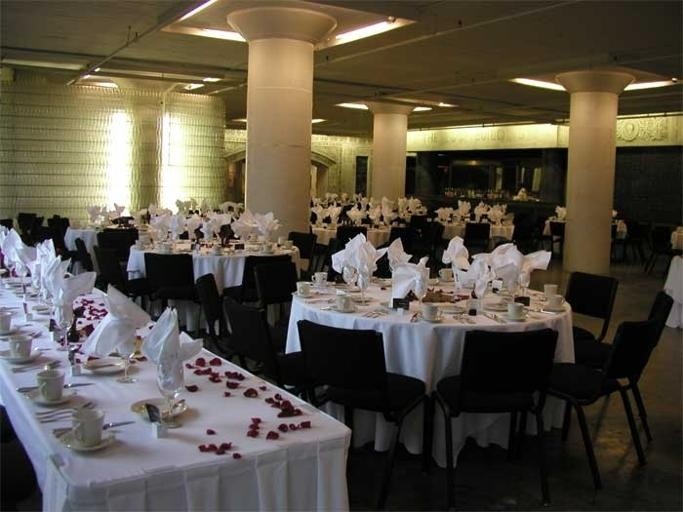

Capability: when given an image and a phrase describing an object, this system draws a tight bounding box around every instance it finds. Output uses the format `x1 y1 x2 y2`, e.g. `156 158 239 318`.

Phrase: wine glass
5 262 27 288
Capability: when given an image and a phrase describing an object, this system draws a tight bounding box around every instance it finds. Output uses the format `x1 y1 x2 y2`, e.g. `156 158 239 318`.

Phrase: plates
295 268 567 325
0 286 188 453
134 232 293 256
